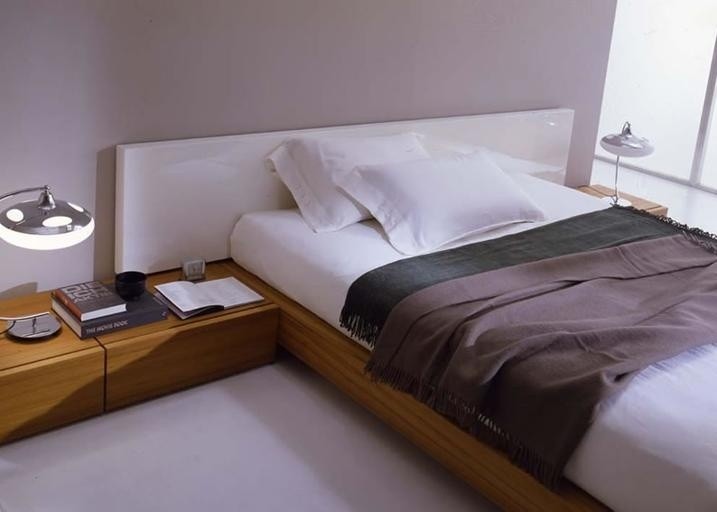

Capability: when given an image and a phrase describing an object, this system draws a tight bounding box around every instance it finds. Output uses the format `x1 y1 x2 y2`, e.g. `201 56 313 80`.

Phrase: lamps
0 185 96 339
598 121 654 207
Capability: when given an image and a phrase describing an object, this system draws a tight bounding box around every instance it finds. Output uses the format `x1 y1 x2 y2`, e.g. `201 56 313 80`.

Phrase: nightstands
571 184 668 221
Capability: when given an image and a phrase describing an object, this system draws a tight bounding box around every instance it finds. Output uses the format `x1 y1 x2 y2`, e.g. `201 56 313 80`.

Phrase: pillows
265 129 548 257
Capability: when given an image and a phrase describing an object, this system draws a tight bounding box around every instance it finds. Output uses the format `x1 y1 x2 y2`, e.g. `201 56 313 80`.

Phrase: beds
227 175 715 510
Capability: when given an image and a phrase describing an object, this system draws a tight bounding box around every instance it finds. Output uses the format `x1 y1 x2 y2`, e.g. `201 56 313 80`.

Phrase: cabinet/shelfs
0 307 279 446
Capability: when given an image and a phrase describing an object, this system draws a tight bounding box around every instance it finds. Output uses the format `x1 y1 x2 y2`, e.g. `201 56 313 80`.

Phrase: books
151 275 265 321
54 280 128 322
50 281 169 340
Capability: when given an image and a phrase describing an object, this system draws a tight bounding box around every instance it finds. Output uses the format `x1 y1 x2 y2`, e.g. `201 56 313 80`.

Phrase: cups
116 270 147 300
